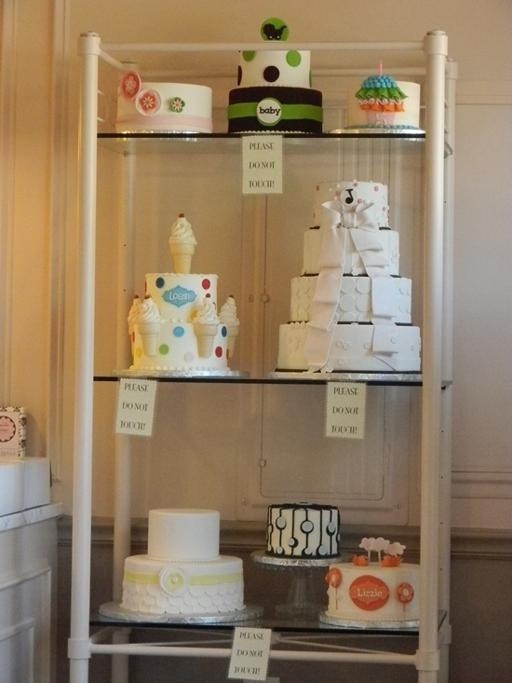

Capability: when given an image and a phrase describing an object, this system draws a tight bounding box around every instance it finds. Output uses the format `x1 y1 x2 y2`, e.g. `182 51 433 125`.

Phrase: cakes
228 17 323 133
120 508 246 614
275 181 421 374
115 70 213 133
344 63 420 129
127 214 240 370
325 537 420 621
264 502 341 559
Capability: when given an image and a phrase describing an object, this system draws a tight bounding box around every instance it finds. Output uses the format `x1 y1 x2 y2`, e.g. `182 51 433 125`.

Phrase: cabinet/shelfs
67 29 457 682
0 504 62 683
